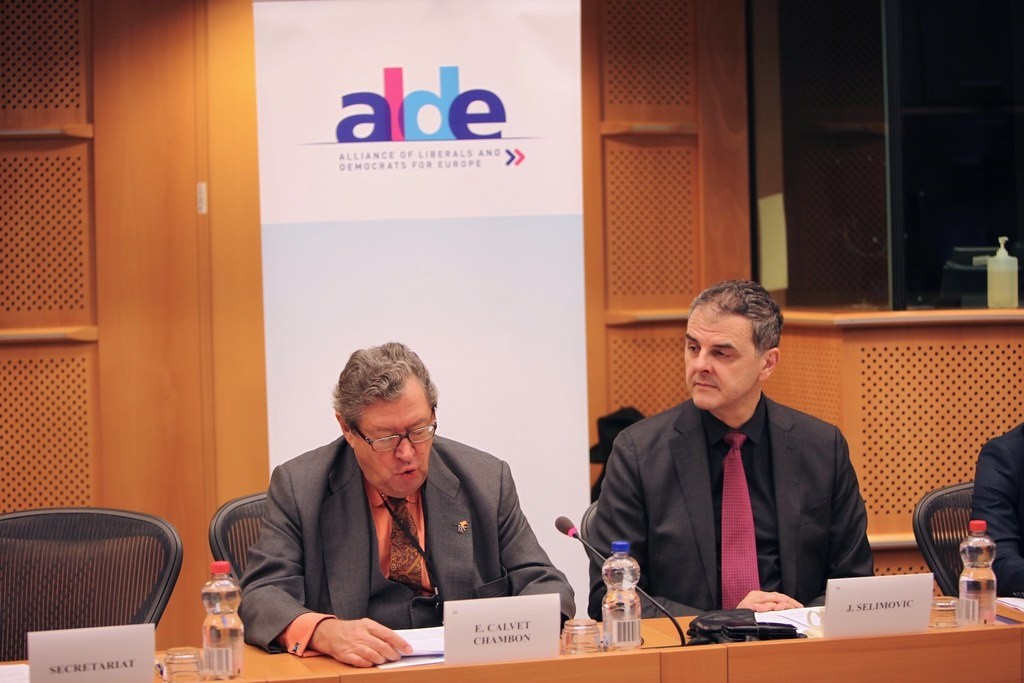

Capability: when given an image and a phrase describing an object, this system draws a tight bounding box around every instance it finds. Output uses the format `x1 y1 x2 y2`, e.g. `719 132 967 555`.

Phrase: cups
165 647 203 683
930 596 959 629
559 618 601 653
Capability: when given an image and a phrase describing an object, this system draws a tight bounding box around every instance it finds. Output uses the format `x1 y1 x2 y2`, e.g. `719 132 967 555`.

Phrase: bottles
957 521 997 628
601 540 642 651
201 561 244 679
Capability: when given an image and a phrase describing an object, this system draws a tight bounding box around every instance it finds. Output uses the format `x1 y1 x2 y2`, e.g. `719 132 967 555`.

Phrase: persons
238 342 576 668
971 422 1024 598
587 281 874 622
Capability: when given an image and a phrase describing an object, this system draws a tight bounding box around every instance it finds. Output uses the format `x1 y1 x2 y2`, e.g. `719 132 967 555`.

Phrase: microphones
555 516 686 647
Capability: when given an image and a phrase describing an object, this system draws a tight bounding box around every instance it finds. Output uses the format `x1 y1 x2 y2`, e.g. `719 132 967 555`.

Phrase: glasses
347 407 442 453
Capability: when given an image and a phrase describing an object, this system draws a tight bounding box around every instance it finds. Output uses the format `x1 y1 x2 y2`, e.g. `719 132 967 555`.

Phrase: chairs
912 480 974 599
0 507 184 662
208 491 268 583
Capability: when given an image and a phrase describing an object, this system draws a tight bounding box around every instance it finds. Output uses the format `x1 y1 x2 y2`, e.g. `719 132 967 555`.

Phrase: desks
641 600 1024 683
0 600 728 683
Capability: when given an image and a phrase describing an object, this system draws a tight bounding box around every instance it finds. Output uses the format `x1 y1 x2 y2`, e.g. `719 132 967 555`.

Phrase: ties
387 501 421 598
720 431 762 610
